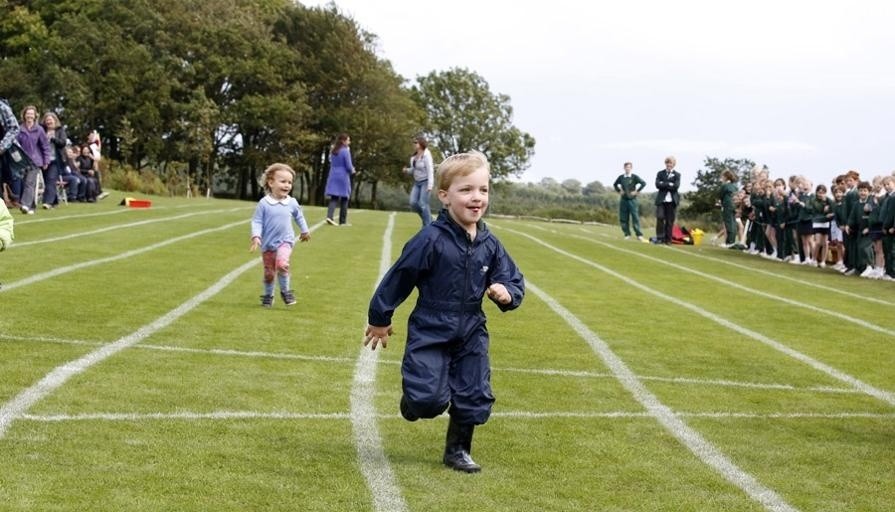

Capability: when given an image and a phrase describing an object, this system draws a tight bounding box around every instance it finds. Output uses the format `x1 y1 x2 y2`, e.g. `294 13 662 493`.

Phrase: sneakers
807 260 817 267
820 262 827 267
860 265 895 281
258 293 274 309
831 260 854 276
801 257 810 264
281 290 296 306
21 205 30 212
43 203 52 209
624 235 629 240
789 255 800 264
718 242 789 260
97 192 110 200
637 235 649 243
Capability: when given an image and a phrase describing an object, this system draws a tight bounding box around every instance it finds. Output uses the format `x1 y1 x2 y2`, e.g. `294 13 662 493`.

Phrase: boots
396 395 416 419
442 419 480 471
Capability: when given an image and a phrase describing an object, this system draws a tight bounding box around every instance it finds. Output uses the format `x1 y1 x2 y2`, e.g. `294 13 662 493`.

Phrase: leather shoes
666 240 672 247
68 196 77 202
341 223 351 227
326 218 338 227
79 196 87 202
654 239 664 244
87 196 96 202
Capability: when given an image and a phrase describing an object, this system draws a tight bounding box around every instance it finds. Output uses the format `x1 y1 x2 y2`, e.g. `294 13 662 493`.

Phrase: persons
365 150 527 476
0 97 20 208
764 179 786 258
60 131 110 201
812 183 834 268
612 162 647 242
0 196 18 253
16 104 51 215
829 170 873 276
321 133 357 227
871 176 884 279
790 172 808 264
402 138 435 232
794 176 816 266
882 176 895 281
654 156 678 245
775 187 791 260
715 169 737 249
733 168 774 255
41 110 67 210
785 176 799 264
249 163 311 309
